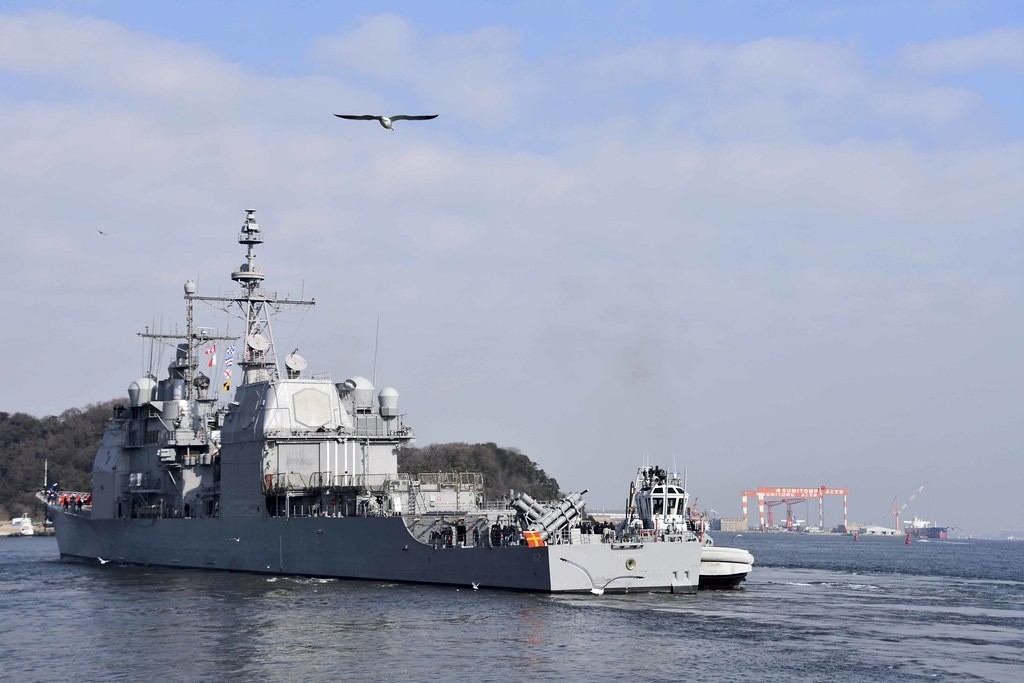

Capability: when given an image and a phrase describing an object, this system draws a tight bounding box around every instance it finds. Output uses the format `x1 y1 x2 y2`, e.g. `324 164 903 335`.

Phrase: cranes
890 477 928 531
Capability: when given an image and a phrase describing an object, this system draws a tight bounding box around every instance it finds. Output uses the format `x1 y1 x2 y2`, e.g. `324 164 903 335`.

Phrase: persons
503 524 516 543
63 495 83 511
473 526 481 546
578 521 614 534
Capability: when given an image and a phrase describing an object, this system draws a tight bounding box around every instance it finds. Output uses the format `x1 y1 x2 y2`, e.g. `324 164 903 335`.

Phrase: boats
36 206 755 595
610 450 713 545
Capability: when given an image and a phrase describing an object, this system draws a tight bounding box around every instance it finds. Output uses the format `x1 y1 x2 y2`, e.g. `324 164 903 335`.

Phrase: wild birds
401 544 409 553
97 557 111 566
472 581 481 590
327 109 446 135
727 533 745 544
228 535 242 544
95 227 110 238
559 557 644 598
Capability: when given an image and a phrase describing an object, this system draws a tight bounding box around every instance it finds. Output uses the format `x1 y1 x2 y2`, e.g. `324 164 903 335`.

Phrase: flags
525 530 544 548
205 343 216 367
222 345 235 392
